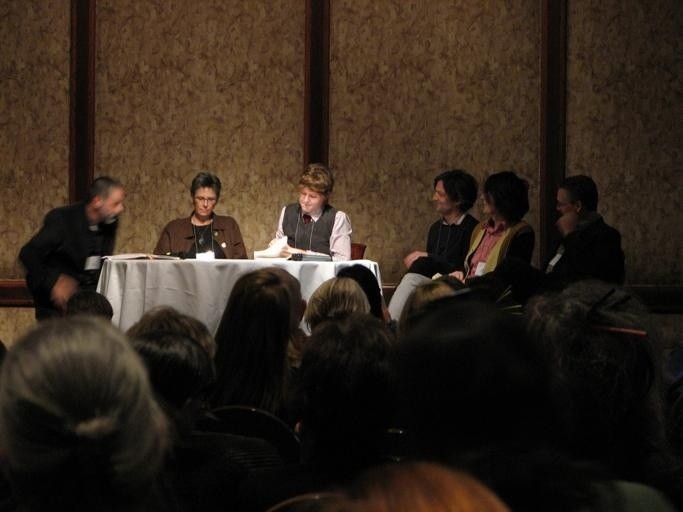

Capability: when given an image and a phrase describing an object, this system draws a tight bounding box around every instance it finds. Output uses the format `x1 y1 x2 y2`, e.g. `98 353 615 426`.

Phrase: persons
19 176 126 321
449 171 536 287
386 168 481 322
1 263 682 511
151 171 250 258
267 163 354 264
512 174 625 305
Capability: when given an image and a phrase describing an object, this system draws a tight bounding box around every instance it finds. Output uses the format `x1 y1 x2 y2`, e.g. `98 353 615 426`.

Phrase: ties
303 215 311 224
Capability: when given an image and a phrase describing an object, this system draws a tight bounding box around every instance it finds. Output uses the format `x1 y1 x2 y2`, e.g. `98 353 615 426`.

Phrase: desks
96 252 382 340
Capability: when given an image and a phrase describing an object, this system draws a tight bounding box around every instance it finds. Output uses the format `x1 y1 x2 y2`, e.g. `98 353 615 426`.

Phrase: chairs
351 243 367 259
495 245 567 316
200 404 304 470
265 491 347 511
